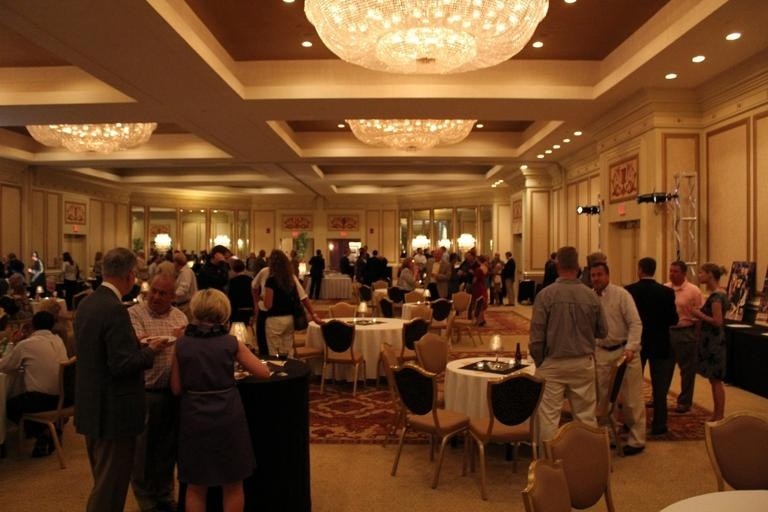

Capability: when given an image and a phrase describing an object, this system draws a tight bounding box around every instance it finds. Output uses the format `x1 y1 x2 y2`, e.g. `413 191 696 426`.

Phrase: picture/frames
281 214 314 231
512 198 522 221
608 153 639 205
65 200 87 226
327 214 360 231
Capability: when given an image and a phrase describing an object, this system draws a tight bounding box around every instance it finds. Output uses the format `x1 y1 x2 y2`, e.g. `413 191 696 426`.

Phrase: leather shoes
675 404 689 412
647 426 666 436
646 396 654 407
623 445 642 456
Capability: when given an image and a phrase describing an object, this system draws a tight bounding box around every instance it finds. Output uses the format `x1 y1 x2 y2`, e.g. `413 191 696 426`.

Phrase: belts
596 340 628 351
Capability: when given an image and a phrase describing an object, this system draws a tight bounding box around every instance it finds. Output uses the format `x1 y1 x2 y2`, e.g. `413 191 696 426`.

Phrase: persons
1 244 104 462
528 244 647 463
610 257 728 433
729 264 751 317
339 245 516 327
72 246 325 511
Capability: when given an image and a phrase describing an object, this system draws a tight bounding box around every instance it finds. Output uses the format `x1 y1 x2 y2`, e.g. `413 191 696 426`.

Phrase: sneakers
32 440 55 456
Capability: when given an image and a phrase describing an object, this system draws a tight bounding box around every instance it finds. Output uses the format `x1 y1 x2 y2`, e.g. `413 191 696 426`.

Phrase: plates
140 335 177 345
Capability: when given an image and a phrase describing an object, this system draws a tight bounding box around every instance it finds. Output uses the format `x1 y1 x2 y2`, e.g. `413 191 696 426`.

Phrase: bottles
514 342 521 369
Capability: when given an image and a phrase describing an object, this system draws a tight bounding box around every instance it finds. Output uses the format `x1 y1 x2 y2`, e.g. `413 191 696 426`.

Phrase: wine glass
276 347 289 376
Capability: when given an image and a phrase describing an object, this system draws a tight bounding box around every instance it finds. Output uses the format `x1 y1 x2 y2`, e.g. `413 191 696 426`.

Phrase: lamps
303 0 549 76
577 205 599 215
343 119 478 150
636 191 680 206
25 121 159 153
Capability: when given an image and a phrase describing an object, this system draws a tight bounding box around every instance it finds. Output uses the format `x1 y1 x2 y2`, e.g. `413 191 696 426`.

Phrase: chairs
292 279 491 404
522 452 572 511
470 371 549 501
540 419 617 511
703 408 768 492
0 277 144 470
386 361 471 490
563 352 631 470
